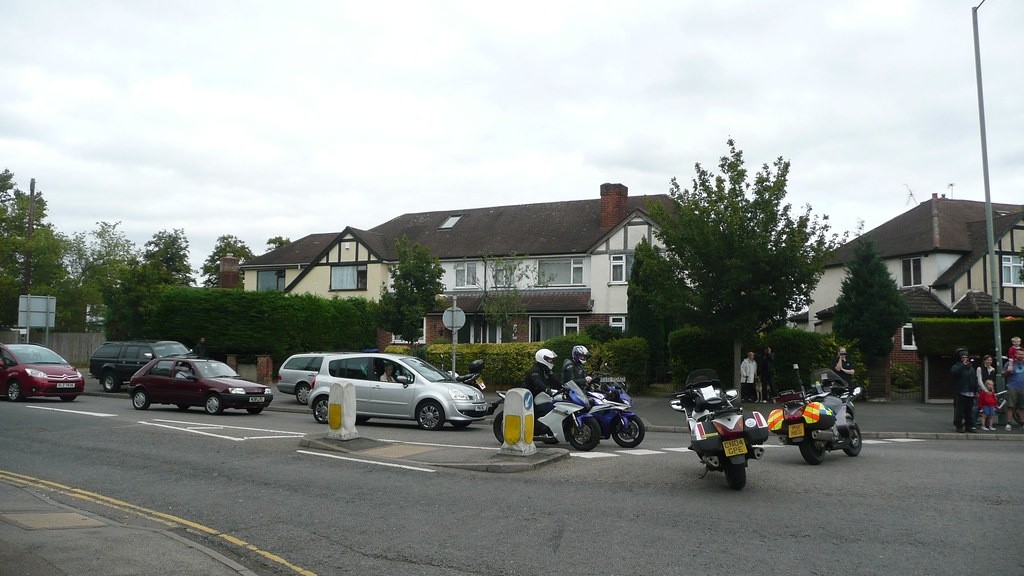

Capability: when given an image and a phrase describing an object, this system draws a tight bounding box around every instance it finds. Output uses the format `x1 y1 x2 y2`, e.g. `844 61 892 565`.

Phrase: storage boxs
468 359 484 373
802 402 837 431
767 408 788 435
742 410 769 444
691 421 723 452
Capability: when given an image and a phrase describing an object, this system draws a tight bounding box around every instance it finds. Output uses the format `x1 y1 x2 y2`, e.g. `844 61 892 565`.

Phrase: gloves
586 376 592 383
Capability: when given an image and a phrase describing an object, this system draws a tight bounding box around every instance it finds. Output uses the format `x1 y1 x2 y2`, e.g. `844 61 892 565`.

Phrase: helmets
572 345 590 367
534 348 558 371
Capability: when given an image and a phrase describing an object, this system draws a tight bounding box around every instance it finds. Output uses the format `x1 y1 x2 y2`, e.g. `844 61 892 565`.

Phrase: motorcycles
668 367 769 489
550 371 646 449
493 379 601 451
440 353 486 390
768 363 863 464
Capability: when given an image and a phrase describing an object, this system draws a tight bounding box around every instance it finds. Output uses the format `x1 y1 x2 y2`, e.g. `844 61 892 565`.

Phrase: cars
308 353 487 431
129 357 273 415
0 343 86 401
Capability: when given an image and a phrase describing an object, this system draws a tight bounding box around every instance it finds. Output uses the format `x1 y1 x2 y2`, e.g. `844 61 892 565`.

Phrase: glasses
839 353 847 355
961 357 968 358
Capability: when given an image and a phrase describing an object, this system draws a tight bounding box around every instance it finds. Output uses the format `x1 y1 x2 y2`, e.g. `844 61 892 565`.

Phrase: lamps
345 243 350 249
513 323 518 333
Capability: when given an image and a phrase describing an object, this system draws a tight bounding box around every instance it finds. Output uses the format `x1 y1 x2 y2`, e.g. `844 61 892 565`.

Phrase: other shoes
980 426 989 431
956 428 964 433
1005 423 1012 431
965 425 978 433
988 426 996 430
971 426 977 429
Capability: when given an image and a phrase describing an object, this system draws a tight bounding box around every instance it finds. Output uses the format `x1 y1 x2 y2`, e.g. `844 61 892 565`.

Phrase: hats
961 351 968 356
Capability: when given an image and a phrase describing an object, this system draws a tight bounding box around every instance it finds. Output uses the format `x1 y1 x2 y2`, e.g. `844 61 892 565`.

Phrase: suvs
277 353 326 405
90 339 199 393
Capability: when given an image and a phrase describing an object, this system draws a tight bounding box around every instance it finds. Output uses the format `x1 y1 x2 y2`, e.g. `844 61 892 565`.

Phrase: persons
520 348 563 406
759 347 777 404
175 362 194 379
380 364 396 382
1008 336 1024 374
561 345 592 400
978 380 997 431
976 355 995 392
832 348 855 388
951 351 977 433
1001 349 1024 431
741 352 758 403
